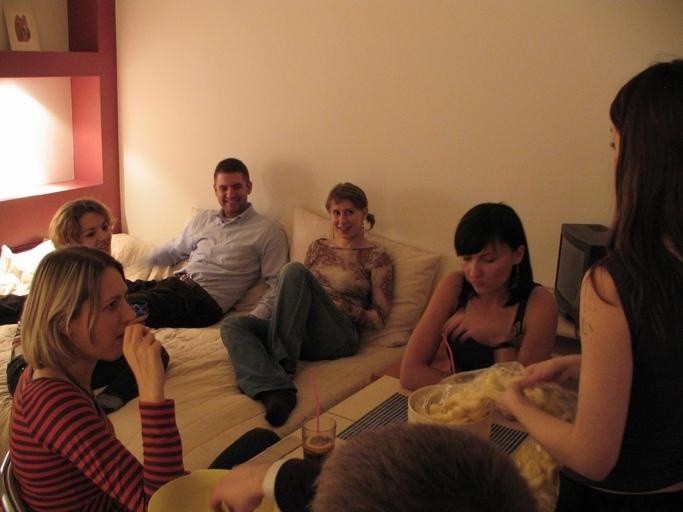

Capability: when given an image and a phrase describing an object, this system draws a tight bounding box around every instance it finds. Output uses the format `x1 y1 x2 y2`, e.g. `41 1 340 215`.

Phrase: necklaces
64 369 103 417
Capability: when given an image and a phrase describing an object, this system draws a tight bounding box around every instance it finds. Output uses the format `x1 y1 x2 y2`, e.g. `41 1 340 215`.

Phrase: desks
232 371 530 511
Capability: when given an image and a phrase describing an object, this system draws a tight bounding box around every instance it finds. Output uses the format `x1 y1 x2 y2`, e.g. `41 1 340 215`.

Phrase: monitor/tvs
553 224 611 330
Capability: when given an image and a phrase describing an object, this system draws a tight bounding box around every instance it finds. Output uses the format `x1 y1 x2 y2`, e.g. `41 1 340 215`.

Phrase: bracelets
493 341 514 351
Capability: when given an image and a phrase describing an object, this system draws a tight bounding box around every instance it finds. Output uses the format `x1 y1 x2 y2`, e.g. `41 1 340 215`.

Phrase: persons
8 246 280 512
0 159 288 329
6 198 169 415
495 59 683 512
220 182 395 427
399 202 559 391
209 422 538 512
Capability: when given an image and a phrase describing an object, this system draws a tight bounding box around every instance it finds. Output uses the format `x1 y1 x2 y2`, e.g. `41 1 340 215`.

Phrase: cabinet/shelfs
1 2 121 253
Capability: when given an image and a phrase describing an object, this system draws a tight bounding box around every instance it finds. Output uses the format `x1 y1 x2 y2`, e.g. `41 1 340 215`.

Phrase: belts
179 273 222 317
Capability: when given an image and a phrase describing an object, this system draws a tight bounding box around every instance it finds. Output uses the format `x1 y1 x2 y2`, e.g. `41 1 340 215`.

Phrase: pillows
290 204 441 349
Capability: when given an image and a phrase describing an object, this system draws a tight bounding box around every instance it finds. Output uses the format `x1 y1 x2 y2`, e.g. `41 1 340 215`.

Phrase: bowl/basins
407 384 492 441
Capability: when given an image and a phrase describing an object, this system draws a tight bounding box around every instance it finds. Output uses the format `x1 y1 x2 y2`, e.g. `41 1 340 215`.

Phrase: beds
1 323 405 476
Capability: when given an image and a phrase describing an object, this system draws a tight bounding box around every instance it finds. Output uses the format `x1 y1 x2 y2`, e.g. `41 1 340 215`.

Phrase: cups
301 415 336 462
